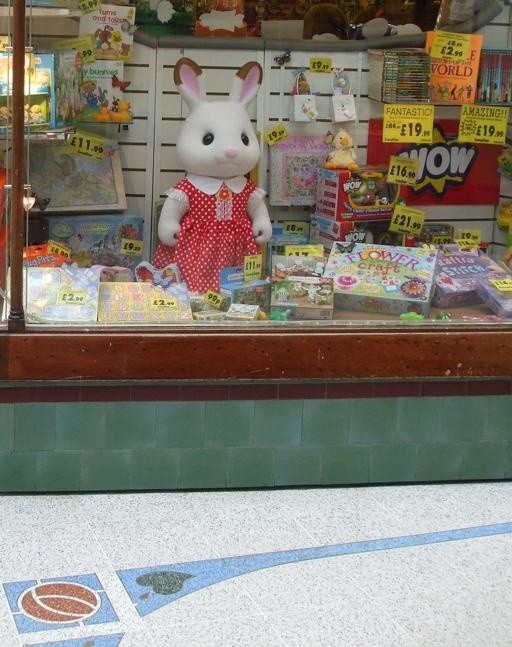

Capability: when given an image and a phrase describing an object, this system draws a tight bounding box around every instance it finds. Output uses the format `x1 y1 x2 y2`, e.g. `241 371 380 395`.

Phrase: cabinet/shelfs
0 303 512 494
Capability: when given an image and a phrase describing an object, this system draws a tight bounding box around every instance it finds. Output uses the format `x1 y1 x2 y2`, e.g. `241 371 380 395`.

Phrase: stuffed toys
326 129 359 171
295 75 310 96
332 72 350 96
0 103 44 127
134 58 274 294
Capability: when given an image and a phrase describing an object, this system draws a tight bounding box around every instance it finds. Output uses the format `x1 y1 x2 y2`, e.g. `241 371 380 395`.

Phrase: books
368 29 511 107
